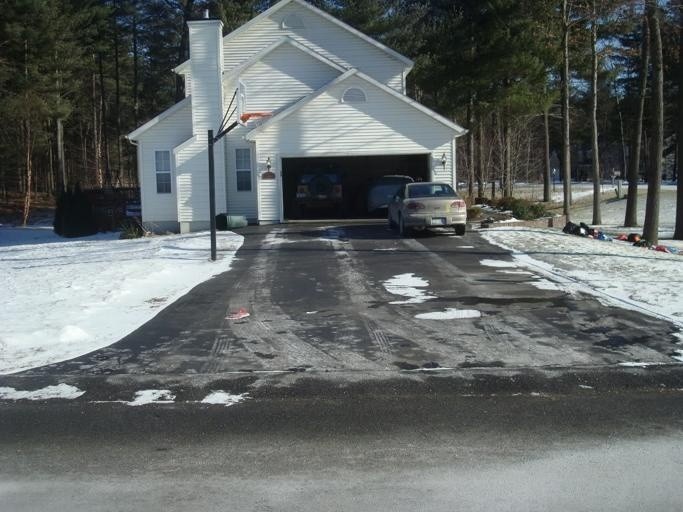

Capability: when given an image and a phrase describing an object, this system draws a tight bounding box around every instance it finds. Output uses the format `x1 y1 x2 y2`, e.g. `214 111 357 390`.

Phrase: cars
366 173 414 216
386 179 467 236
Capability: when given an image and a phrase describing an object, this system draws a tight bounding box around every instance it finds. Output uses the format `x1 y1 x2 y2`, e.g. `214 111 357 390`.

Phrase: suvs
296 161 344 216
